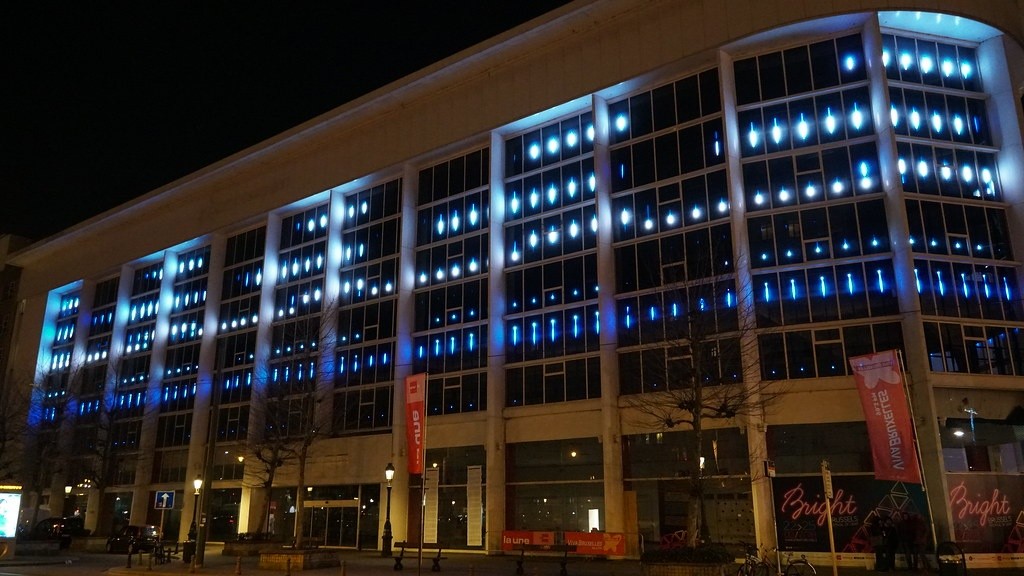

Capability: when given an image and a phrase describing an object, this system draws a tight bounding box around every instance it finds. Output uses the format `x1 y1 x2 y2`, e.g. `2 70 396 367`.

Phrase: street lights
52 485 73 541
700 456 713 547
381 462 396 555
186 475 203 541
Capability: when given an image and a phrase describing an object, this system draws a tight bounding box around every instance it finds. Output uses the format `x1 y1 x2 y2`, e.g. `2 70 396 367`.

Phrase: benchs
510 544 578 574
391 540 449 571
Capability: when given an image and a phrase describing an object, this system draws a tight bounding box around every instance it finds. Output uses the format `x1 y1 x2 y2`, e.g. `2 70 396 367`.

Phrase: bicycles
750 543 817 576
736 539 764 576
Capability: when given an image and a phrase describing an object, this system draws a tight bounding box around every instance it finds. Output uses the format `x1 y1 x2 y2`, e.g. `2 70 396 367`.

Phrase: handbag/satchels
871 535 888 547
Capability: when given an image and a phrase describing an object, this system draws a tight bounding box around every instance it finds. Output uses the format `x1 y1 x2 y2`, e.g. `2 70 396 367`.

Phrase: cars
105 524 164 554
28 516 84 539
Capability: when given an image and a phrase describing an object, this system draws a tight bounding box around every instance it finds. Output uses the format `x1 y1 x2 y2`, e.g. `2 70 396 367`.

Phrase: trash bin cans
874 544 896 572
182 540 196 563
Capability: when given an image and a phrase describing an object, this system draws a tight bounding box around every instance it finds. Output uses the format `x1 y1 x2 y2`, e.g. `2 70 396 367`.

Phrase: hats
871 516 881 522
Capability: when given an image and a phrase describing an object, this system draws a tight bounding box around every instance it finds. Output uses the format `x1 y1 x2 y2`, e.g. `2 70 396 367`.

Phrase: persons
189 524 198 557
900 512 919 572
870 516 887 573
882 517 898 571
916 513 931 576
